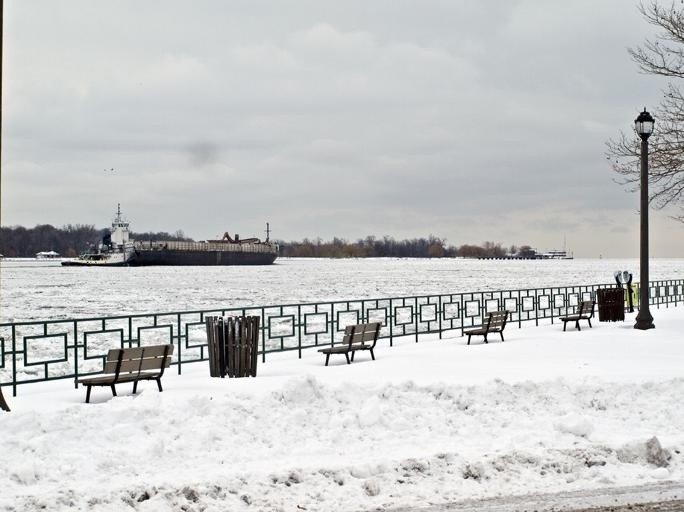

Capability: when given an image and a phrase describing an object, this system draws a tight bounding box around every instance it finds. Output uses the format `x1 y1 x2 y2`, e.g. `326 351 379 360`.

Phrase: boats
36 251 59 261
61 203 279 265
0 254 3 260
505 246 573 259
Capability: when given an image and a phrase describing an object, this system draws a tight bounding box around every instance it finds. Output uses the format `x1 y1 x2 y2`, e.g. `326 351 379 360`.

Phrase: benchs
462 310 509 345
317 322 382 366
75 344 175 403
559 301 596 331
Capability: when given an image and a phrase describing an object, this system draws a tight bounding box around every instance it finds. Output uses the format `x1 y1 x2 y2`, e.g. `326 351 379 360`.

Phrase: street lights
634 113 655 330
